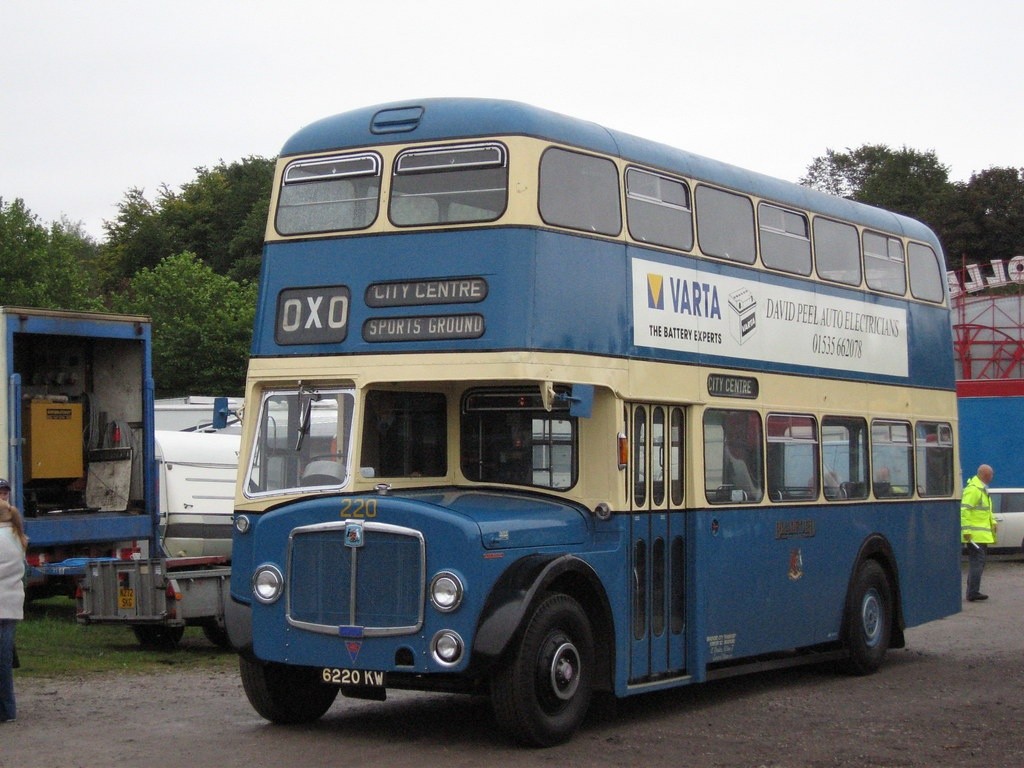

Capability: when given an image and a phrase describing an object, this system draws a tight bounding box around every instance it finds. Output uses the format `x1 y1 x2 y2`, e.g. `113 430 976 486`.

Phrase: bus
207 97 968 748
74 385 679 655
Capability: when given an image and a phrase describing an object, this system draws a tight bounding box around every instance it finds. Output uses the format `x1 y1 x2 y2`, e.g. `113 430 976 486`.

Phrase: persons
0 478 29 670
0 499 28 723
960 464 999 600
876 467 906 493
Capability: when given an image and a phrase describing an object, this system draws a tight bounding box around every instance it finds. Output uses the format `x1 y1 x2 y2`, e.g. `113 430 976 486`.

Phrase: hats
0 479 11 491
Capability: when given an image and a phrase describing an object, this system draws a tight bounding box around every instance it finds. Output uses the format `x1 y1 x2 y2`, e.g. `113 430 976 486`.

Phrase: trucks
2 308 163 600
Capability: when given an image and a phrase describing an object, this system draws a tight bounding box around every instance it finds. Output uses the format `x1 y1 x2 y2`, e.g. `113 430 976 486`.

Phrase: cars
988 488 1024 551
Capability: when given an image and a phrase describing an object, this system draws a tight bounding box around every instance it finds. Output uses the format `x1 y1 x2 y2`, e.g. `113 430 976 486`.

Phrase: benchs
716 481 926 501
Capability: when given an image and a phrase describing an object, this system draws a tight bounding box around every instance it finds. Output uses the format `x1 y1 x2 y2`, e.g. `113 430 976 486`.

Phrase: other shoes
0 716 17 723
965 592 989 600
